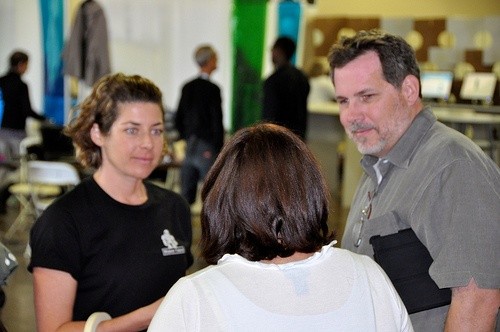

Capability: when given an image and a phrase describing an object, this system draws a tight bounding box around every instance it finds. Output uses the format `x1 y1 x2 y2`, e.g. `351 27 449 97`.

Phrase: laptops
417 70 454 102
458 72 497 103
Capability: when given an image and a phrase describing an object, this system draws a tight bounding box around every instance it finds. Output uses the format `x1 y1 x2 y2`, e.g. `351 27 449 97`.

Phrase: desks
142 161 206 193
307 99 500 250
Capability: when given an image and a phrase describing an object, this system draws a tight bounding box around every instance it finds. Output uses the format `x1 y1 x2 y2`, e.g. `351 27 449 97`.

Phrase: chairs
3 117 79 243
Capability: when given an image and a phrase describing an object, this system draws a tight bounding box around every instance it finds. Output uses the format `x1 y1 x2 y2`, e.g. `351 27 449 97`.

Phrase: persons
324 30 500 332
146 122 415 332
26 72 193 332
0 32 310 206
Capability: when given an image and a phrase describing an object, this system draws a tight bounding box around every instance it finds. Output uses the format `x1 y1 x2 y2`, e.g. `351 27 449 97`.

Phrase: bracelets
83 312 112 332
